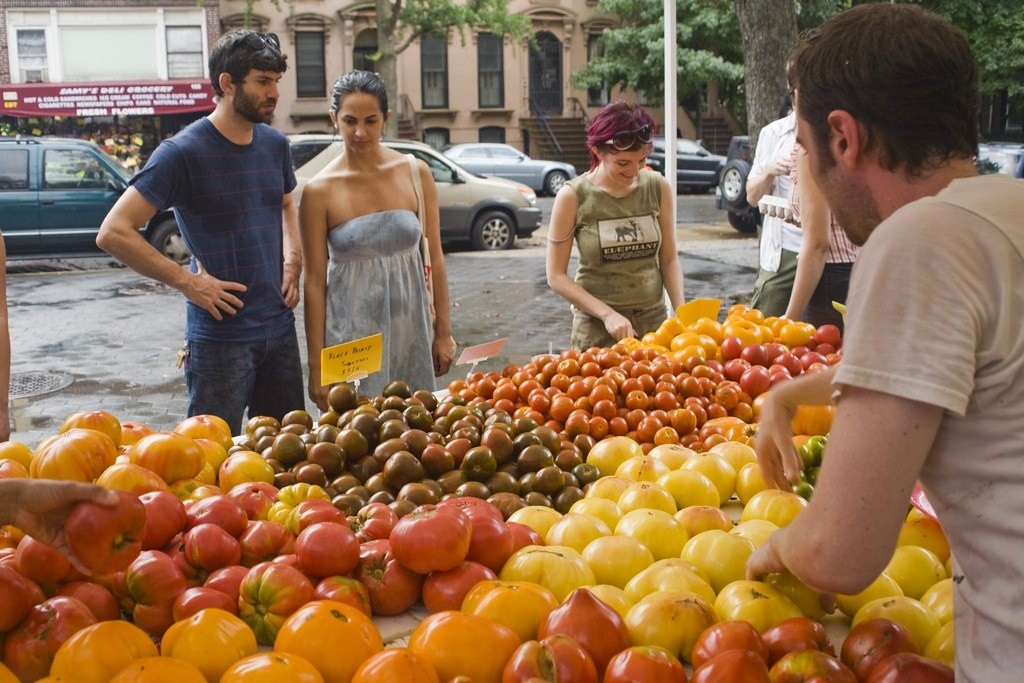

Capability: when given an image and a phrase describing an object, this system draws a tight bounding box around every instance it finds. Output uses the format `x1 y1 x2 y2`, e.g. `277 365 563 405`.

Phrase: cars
432 142 578 198
282 134 543 252
645 136 722 196
0 135 193 270
974 141 1024 182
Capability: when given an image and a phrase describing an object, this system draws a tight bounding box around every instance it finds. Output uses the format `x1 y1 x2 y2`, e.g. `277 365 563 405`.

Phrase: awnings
0 79 220 117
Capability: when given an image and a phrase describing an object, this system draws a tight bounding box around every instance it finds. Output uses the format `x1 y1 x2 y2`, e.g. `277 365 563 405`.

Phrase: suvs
714 135 763 233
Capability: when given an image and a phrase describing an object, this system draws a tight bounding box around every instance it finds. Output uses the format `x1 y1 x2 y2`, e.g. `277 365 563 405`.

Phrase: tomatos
0 301 962 683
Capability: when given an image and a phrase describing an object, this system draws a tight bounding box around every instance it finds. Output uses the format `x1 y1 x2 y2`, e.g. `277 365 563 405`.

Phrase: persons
746 28 863 339
298 71 456 414
546 100 685 354
746 2 1024 683
96 30 305 437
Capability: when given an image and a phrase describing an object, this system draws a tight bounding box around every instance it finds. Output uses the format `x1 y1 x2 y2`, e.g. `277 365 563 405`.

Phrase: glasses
230 32 280 52
599 122 654 150
